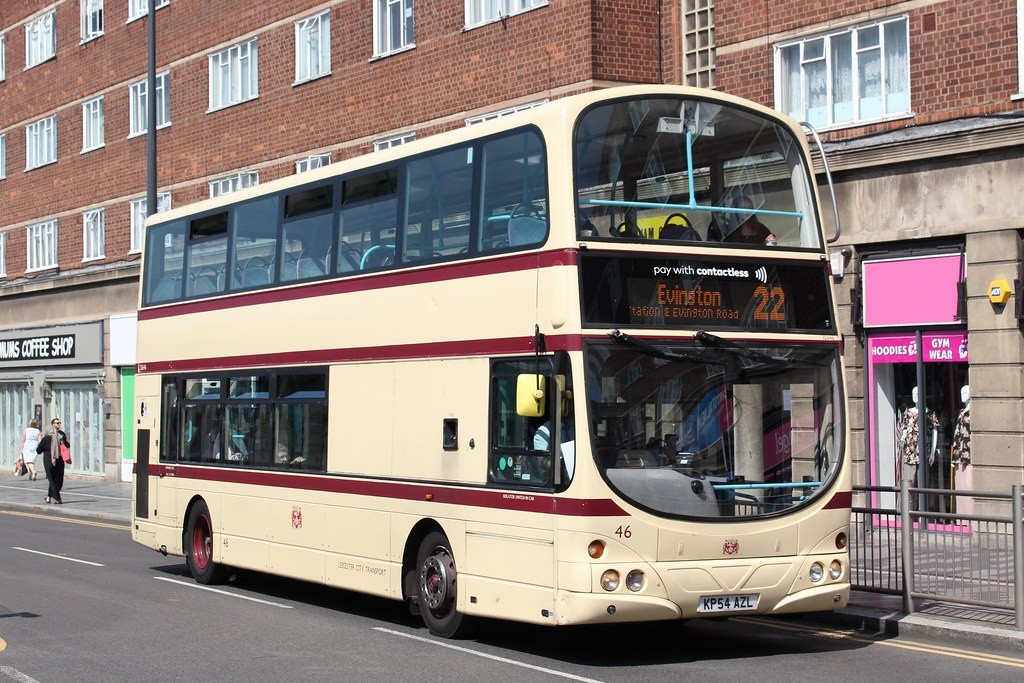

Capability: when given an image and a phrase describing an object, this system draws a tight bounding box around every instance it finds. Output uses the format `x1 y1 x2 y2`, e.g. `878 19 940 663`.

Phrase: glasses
57 422 61 424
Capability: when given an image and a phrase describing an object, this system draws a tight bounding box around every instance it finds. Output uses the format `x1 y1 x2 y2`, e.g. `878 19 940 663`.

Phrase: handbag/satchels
15 454 28 476
60 444 72 464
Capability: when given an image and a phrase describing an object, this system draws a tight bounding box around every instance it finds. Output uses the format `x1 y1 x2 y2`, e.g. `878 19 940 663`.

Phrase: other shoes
54 501 62 504
33 472 37 481
29 473 33 480
44 497 51 503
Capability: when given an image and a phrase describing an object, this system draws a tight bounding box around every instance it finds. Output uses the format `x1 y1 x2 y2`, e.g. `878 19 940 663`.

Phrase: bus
129 83 854 641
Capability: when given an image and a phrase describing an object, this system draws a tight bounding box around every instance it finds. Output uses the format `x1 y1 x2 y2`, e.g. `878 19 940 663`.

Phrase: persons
533 390 602 483
710 196 777 246
212 409 253 461
19 418 42 481
35 425 63 504
51 419 70 492
278 442 306 464
951 385 972 526
897 386 938 524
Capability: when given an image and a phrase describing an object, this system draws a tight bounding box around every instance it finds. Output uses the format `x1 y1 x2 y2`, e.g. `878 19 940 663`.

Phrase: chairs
148 200 731 303
